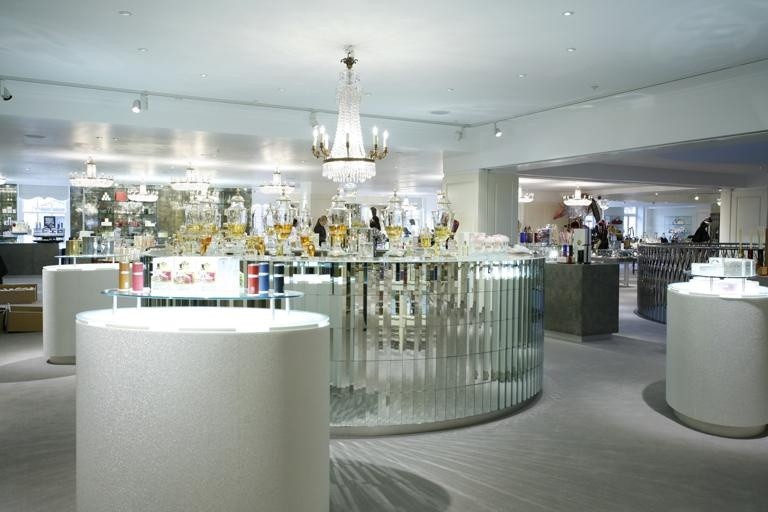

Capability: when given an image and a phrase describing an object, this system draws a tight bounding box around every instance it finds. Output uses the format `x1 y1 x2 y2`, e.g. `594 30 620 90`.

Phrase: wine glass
456 231 509 260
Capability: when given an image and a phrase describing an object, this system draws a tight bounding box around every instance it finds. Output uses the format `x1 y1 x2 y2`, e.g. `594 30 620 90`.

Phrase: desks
100 287 305 310
54 254 123 264
544 262 620 346
590 256 637 287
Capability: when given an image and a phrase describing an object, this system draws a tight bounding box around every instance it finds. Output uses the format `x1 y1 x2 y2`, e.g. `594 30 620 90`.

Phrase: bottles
384 190 406 256
420 195 454 257
247 262 269 296
328 188 349 256
265 187 315 257
118 261 131 290
225 187 248 255
272 264 285 294
184 199 222 254
132 260 144 292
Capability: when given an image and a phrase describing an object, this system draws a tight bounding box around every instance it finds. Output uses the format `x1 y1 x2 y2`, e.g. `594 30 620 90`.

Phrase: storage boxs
0 282 43 332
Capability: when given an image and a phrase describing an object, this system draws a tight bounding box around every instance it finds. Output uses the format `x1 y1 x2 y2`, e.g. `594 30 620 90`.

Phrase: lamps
519 186 536 203
494 121 503 137
130 90 153 115
561 185 594 207
69 154 298 195
0 78 13 101
310 45 391 185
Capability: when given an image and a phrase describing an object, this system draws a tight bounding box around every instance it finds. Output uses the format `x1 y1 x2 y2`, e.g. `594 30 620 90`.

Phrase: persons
403 227 411 235
291 219 299 237
409 219 419 232
313 215 329 245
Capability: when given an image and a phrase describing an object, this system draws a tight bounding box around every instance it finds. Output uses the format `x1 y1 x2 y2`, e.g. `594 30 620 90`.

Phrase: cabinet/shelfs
0 182 19 243
666 282 768 438
74 306 331 512
41 263 120 364
